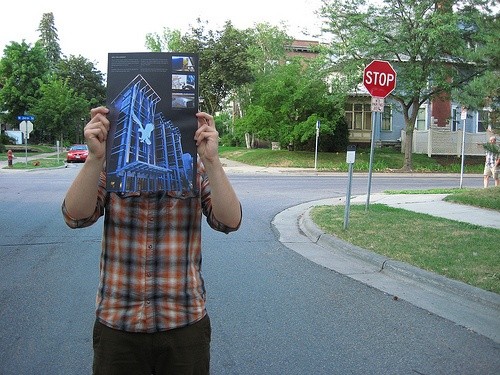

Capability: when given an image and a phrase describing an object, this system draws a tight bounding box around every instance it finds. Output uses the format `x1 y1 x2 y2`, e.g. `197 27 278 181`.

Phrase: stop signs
363 60 397 98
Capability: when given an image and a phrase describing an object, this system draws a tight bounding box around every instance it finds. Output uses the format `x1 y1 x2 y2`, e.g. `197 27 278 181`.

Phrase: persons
484 136 499 188
61 106 242 375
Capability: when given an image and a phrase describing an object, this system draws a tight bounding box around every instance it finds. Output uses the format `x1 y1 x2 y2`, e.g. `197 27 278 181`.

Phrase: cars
66 144 89 163
